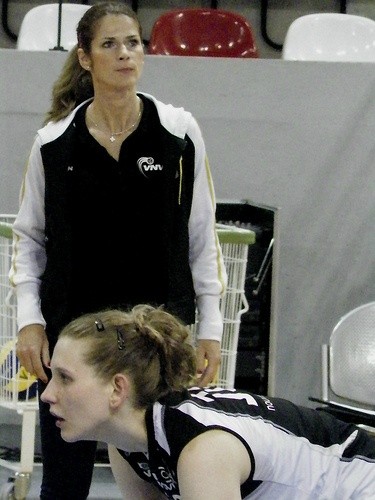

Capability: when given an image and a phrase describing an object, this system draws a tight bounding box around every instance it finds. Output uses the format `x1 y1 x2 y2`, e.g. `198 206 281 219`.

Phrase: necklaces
88 99 142 142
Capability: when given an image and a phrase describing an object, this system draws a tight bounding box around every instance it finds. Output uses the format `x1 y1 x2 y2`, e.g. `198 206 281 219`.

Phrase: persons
7 2 227 500
40 303 375 500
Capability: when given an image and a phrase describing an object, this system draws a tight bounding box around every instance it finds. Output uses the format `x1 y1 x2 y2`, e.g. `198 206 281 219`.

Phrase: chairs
15 4 89 51
281 13 375 62
321 303 375 417
147 9 258 59
